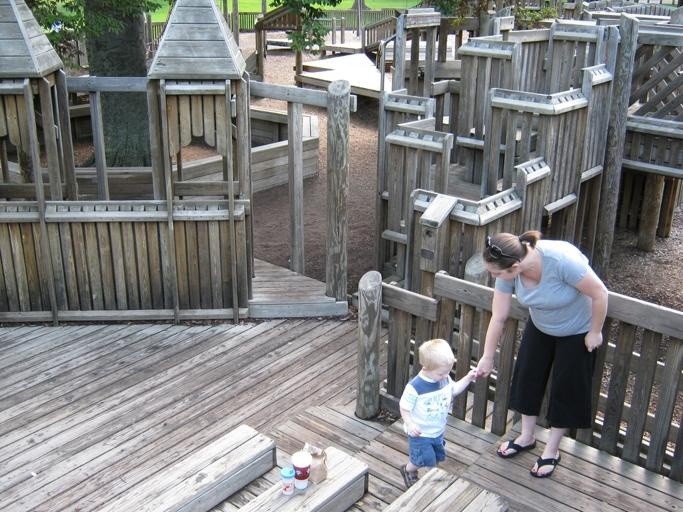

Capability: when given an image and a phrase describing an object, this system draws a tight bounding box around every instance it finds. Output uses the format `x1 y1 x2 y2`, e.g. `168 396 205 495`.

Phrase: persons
471 229 609 478
391 338 480 488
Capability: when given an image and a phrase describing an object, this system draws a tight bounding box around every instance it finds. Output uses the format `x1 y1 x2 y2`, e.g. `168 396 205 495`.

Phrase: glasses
483 234 522 264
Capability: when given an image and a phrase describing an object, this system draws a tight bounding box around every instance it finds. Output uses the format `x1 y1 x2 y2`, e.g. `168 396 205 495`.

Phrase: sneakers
399 463 419 488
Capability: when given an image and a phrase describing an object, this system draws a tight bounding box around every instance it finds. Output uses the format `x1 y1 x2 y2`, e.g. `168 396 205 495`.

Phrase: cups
309 449 328 485
291 450 313 490
281 467 296 496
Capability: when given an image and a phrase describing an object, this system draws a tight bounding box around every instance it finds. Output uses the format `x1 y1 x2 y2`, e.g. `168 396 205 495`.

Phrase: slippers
529 449 562 478
497 434 537 459
